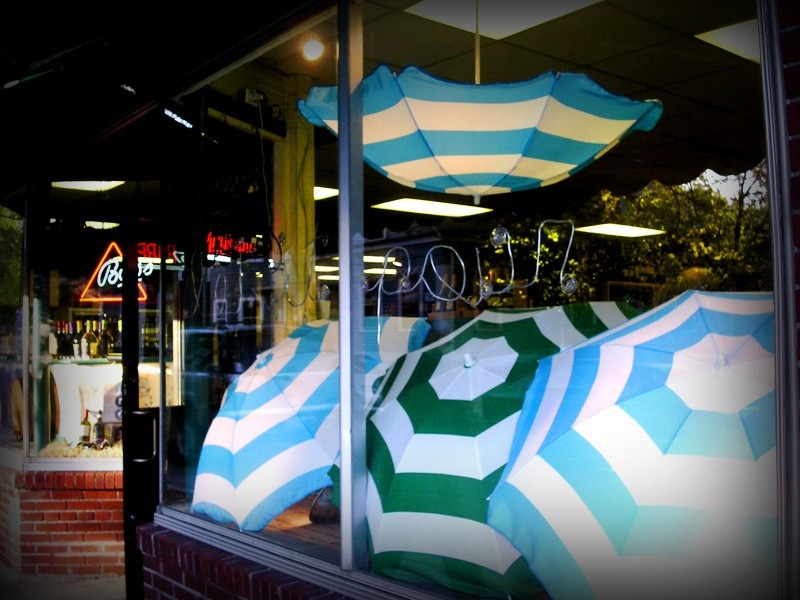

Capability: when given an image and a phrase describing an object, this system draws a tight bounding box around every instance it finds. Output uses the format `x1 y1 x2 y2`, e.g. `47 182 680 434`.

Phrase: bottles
79 410 90 446
93 410 104 450
55 314 160 360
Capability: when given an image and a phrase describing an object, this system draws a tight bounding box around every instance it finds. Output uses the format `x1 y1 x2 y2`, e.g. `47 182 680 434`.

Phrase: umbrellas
191 316 432 533
297 0 663 205
325 300 642 600
485 288 778 600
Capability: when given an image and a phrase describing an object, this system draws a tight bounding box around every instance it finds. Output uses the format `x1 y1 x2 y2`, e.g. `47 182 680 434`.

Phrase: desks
48 356 124 447
